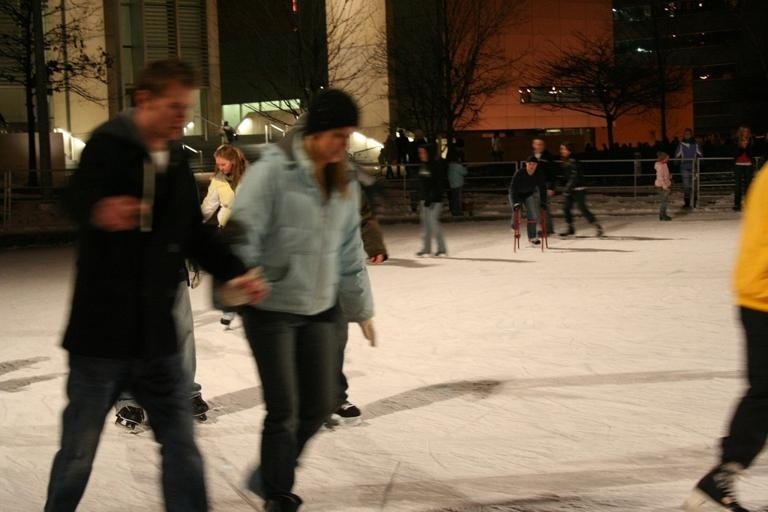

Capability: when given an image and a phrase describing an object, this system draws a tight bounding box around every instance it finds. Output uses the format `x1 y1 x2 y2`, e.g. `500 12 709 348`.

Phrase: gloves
541 202 547 209
514 202 520 208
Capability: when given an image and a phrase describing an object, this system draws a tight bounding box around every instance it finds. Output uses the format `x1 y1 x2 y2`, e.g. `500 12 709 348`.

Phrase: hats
308 87 357 133
526 156 537 162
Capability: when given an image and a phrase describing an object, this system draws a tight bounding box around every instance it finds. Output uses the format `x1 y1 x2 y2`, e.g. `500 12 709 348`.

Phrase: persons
615 140 660 149
222 121 238 144
491 132 505 161
333 187 388 419
655 126 768 220
199 143 251 325
43 60 270 512
382 127 470 256
509 138 604 244
212 90 378 512
114 260 209 425
696 161 768 512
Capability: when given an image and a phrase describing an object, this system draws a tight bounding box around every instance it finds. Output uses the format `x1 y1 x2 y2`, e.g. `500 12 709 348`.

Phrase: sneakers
335 401 360 417
733 207 740 210
531 238 540 244
221 311 233 324
512 229 519 237
369 253 387 263
660 215 670 220
249 467 302 504
560 229 573 236
119 406 142 424
698 468 748 512
596 224 603 236
191 396 208 416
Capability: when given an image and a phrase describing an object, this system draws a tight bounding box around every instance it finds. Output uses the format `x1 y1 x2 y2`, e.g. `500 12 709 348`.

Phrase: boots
683 196 697 208
